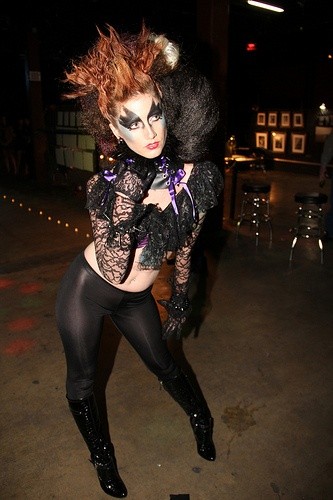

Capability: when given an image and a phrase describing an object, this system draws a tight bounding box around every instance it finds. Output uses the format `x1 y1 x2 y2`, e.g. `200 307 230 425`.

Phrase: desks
224 154 269 221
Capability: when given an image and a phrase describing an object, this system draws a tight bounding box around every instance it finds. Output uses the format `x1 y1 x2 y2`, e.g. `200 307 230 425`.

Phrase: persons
55 20 226 498
319 129 333 187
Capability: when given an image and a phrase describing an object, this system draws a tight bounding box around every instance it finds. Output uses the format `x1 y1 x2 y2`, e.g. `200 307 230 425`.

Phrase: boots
155 363 217 460
65 391 127 498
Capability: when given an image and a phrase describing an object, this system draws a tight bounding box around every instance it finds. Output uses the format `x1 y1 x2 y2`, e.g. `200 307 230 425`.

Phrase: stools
233 182 273 248
289 191 328 265
